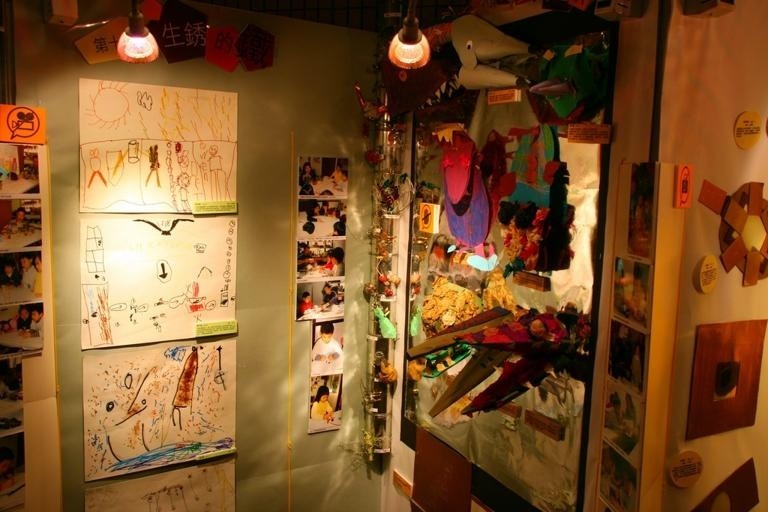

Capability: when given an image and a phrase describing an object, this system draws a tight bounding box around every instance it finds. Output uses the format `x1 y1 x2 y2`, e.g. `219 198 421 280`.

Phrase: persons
1 206 35 238
0 303 44 355
299 284 338 313
0 252 41 301
297 246 344 276
310 387 334 421
299 162 348 195
298 204 346 236
312 323 343 362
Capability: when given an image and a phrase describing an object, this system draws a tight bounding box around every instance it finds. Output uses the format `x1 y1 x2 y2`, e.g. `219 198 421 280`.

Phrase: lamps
115 0 160 65
388 0 432 72
